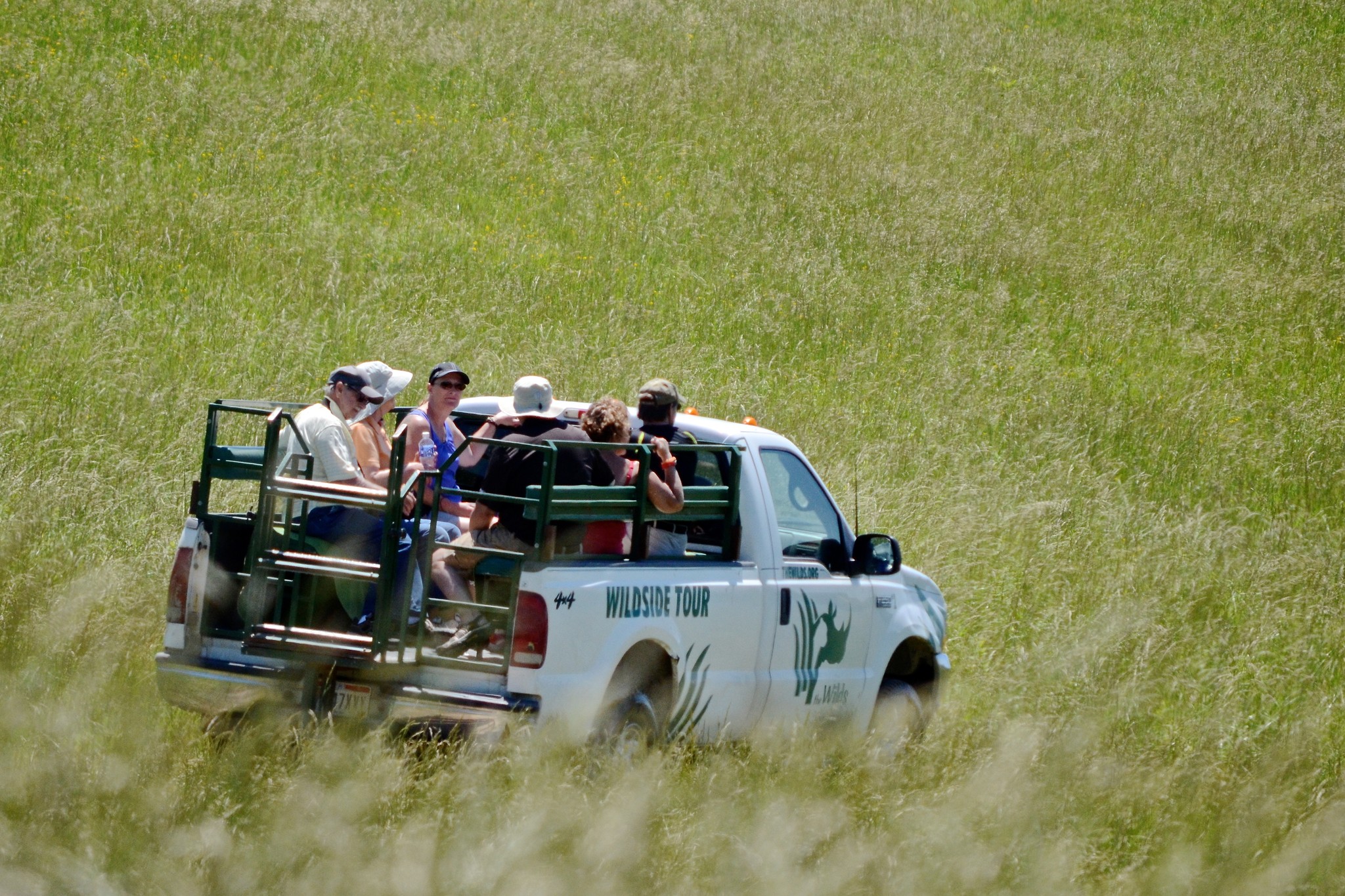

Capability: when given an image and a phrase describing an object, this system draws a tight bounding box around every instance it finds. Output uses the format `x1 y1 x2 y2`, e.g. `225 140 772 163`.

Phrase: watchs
486 417 499 429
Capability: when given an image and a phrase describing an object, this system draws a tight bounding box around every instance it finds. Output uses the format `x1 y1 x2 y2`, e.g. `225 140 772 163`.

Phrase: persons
428 376 617 657
393 362 713 561
278 365 443 642
344 360 461 600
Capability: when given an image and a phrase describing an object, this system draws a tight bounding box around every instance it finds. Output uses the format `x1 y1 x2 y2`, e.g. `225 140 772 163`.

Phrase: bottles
418 432 434 471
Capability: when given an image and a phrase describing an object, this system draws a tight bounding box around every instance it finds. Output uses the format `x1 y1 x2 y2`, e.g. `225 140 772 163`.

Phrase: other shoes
424 616 460 634
488 632 507 649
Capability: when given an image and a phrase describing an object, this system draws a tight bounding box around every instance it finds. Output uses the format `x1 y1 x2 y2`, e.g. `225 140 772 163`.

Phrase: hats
327 365 384 406
498 376 567 418
638 378 686 405
344 360 412 426
428 363 470 385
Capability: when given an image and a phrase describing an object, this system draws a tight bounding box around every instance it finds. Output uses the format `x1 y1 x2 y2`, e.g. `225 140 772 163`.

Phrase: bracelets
661 456 677 470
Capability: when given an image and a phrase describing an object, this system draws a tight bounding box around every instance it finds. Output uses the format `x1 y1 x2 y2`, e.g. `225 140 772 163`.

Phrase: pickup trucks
155 390 955 770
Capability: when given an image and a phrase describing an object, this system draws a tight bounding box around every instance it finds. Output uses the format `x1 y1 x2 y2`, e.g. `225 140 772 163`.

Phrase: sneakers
436 614 490 653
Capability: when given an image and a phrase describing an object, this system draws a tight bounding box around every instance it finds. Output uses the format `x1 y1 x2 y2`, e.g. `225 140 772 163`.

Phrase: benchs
213 400 464 658
417 437 748 672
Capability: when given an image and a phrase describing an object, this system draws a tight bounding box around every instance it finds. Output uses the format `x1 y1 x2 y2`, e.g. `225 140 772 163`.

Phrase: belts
645 520 687 534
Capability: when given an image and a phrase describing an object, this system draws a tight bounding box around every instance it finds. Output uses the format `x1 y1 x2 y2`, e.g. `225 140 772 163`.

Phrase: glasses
433 381 465 391
672 401 682 410
341 381 369 405
627 428 634 436
